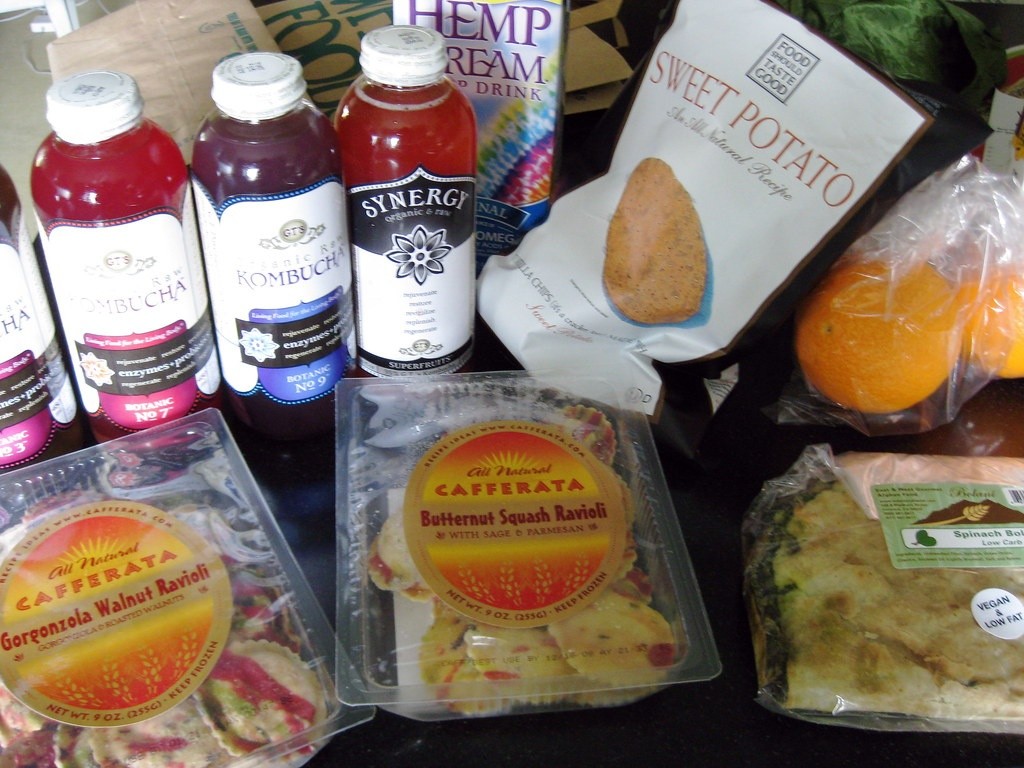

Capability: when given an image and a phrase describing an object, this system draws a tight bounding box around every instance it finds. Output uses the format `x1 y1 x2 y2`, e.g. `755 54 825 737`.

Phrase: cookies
603 158 706 325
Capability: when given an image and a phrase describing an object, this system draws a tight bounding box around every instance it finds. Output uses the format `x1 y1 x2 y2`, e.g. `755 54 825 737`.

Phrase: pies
748 478 1023 722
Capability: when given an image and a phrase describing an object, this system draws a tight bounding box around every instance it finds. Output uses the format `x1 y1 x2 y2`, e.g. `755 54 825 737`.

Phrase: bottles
333 25 476 377
187 51 358 449
30 70 221 451
0 163 86 477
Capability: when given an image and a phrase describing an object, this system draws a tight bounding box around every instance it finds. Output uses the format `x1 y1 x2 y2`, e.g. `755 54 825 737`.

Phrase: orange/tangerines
796 254 1024 412
